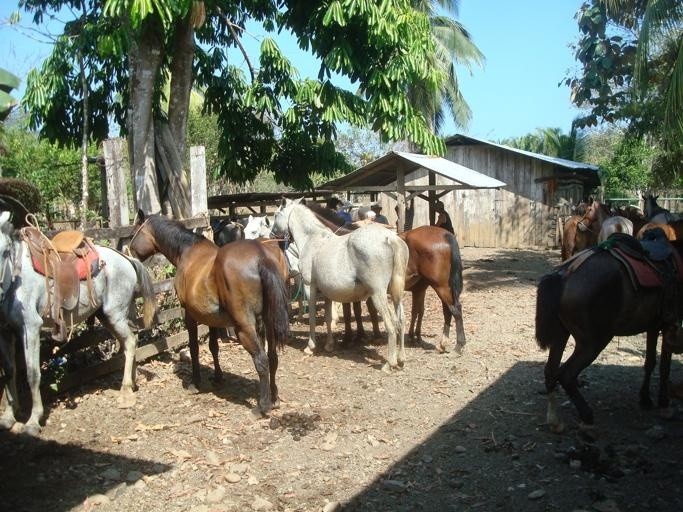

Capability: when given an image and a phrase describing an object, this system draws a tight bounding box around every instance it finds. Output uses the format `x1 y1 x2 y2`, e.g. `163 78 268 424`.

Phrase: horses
534 222 683 434
121 207 294 423
211 196 468 376
560 193 682 261
0 210 159 445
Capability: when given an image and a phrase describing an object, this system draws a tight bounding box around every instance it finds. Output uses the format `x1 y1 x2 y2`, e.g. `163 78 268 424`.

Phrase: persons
328 198 454 237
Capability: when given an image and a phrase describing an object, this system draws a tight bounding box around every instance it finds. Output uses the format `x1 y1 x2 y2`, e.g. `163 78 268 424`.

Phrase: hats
431 201 445 213
371 204 382 211
